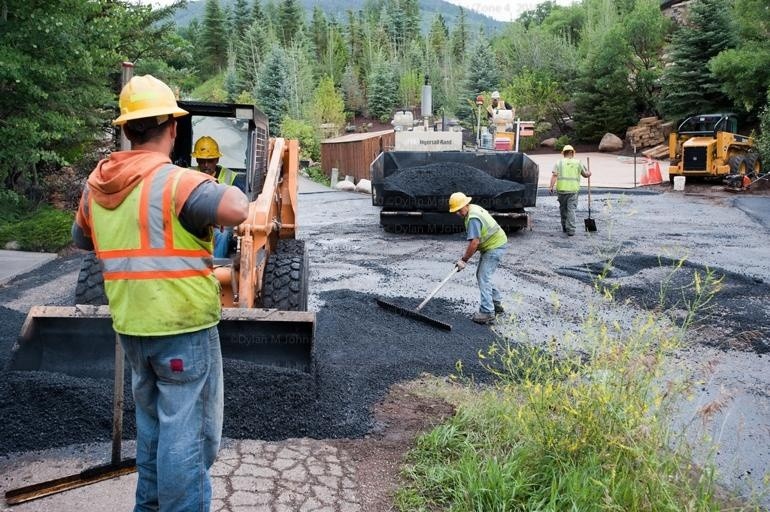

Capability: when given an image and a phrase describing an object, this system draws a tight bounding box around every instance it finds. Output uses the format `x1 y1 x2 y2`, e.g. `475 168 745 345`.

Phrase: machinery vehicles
367 70 541 235
669 113 761 181
1 61 316 401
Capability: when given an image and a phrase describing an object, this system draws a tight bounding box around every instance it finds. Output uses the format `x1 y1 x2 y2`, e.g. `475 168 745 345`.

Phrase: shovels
584 158 597 233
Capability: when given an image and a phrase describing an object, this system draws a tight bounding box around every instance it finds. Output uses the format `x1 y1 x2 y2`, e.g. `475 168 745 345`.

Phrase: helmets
449 192 472 213
112 74 189 127
190 136 223 159
561 144 576 157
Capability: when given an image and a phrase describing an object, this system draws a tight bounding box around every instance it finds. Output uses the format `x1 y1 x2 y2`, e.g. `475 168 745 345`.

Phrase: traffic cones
646 155 655 185
639 162 649 186
654 161 663 185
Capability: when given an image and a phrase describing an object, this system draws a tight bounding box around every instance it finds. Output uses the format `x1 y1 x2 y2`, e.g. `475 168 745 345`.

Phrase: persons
448 191 513 324
182 136 246 258
549 144 592 236
486 90 515 134
70 75 250 511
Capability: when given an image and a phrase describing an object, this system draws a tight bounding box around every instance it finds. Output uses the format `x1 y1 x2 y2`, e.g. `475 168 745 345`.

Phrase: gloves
455 257 468 272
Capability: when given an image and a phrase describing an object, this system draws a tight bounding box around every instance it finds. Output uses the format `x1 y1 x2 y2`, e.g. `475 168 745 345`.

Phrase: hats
491 90 500 99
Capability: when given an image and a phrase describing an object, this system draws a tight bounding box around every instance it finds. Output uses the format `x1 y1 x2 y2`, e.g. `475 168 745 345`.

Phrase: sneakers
495 306 505 313
568 229 575 236
563 223 567 232
473 313 496 322
